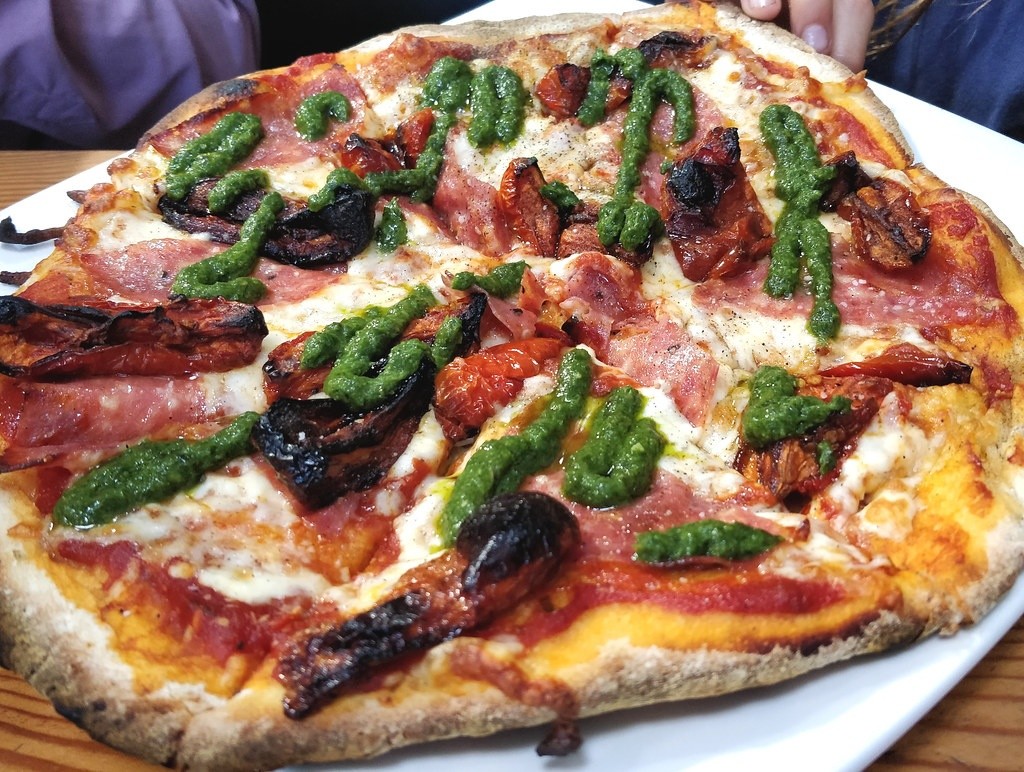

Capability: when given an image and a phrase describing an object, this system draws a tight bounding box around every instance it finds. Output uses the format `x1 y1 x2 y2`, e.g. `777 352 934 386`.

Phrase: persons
729 1 1024 131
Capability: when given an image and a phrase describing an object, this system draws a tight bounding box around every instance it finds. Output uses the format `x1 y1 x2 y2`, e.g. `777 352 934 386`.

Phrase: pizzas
0 0 1024 772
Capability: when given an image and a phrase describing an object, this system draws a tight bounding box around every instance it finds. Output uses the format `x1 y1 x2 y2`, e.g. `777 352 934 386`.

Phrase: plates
0 0 1023 772
